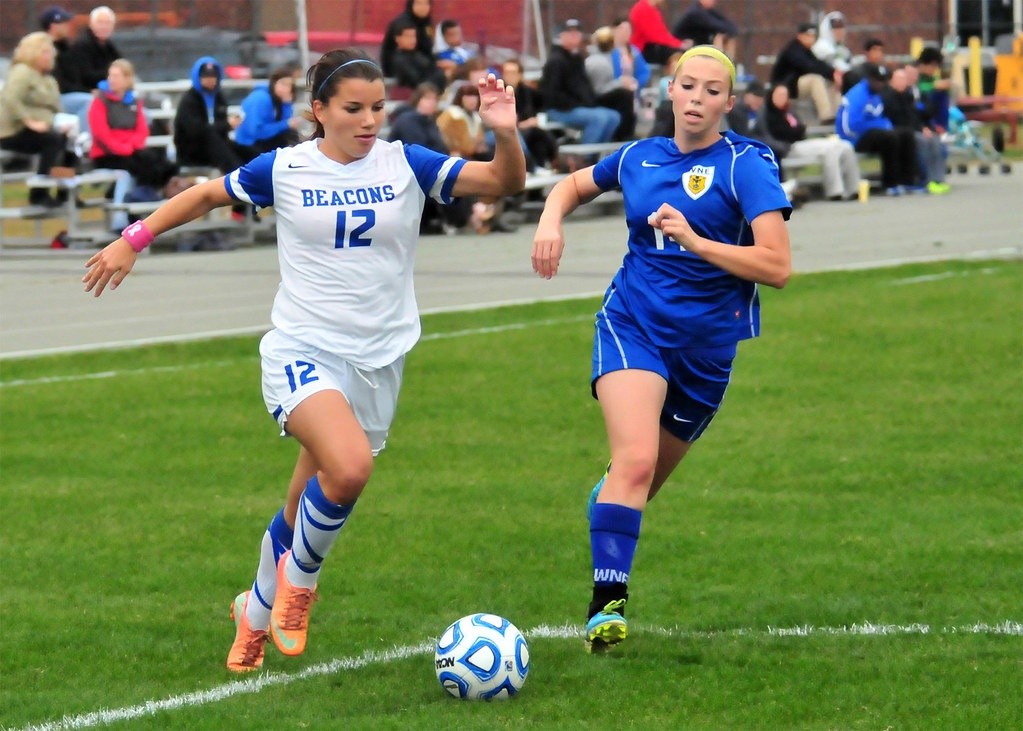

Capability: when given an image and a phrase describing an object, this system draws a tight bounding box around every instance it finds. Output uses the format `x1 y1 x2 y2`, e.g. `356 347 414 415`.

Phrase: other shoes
163 177 193 199
886 183 922 194
55 191 85 207
29 192 59 208
231 202 262 222
926 181 949 195
489 218 518 232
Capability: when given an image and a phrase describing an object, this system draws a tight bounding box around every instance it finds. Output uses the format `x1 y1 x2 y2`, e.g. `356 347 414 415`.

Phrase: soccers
434 614 530 700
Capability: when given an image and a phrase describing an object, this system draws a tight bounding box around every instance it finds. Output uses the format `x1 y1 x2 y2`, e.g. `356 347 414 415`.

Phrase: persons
0 0 951 236
531 45 793 654
82 48 526 673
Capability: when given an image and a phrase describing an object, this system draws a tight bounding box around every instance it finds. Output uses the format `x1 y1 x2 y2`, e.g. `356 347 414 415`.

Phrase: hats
870 64 892 80
39 6 72 32
563 19 581 31
199 62 219 78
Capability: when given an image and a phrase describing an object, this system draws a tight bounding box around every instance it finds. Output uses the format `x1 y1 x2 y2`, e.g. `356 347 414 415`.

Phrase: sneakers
226 592 271 671
584 598 628 654
269 550 317 656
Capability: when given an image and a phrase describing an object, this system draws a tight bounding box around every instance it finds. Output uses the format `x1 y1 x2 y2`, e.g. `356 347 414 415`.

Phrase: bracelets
121 220 155 252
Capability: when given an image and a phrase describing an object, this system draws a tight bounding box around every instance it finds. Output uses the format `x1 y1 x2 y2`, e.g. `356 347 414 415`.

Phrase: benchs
0 71 956 253
